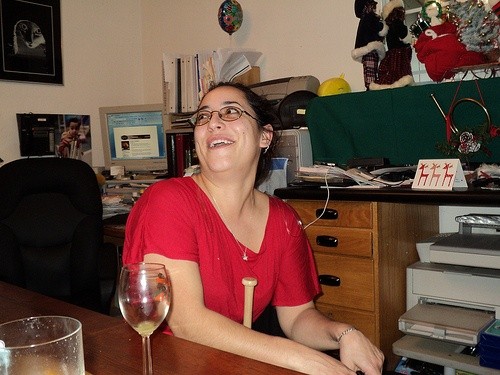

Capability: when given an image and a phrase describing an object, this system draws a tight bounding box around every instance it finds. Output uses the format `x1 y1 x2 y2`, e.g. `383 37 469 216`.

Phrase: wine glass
118 260 172 375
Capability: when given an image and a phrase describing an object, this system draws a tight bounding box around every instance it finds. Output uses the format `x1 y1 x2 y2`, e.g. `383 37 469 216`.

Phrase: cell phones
315 160 338 167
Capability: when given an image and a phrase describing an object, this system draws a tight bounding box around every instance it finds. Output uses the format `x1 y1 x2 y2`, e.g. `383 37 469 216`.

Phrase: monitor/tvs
99 103 170 171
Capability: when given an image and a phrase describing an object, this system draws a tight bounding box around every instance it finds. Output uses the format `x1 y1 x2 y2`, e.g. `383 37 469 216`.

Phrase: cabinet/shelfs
280 203 441 372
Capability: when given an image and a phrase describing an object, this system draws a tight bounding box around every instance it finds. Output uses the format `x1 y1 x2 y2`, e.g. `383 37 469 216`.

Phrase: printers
397 213 500 343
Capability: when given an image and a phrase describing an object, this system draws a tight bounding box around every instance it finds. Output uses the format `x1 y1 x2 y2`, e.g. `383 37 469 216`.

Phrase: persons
60 118 81 155
122 82 385 375
369 0 413 90
418 0 444 26
352 0 389 90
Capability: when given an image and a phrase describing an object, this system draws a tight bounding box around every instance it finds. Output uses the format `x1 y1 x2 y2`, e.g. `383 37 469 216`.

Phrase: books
163 48 262 112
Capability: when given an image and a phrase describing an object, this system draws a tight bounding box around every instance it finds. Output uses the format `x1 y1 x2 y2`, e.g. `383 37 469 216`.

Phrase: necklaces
200 175 253 260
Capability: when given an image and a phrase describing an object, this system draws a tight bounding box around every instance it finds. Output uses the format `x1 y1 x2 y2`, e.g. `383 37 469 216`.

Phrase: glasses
188 106 264 129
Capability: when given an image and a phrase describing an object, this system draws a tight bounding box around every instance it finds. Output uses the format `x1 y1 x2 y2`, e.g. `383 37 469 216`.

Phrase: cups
0 316 86 375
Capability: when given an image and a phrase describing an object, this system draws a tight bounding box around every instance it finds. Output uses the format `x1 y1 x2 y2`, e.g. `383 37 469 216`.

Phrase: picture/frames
0 0 64 83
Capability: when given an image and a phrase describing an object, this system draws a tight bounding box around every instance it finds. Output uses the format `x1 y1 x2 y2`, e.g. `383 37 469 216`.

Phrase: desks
271 183 500 372
0 283 320 374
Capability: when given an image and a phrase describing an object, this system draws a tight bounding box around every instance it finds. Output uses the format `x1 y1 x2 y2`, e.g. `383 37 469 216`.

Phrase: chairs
0 156 107 315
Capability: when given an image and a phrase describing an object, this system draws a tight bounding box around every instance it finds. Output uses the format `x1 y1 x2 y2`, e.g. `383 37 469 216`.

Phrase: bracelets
337 327 356 342
